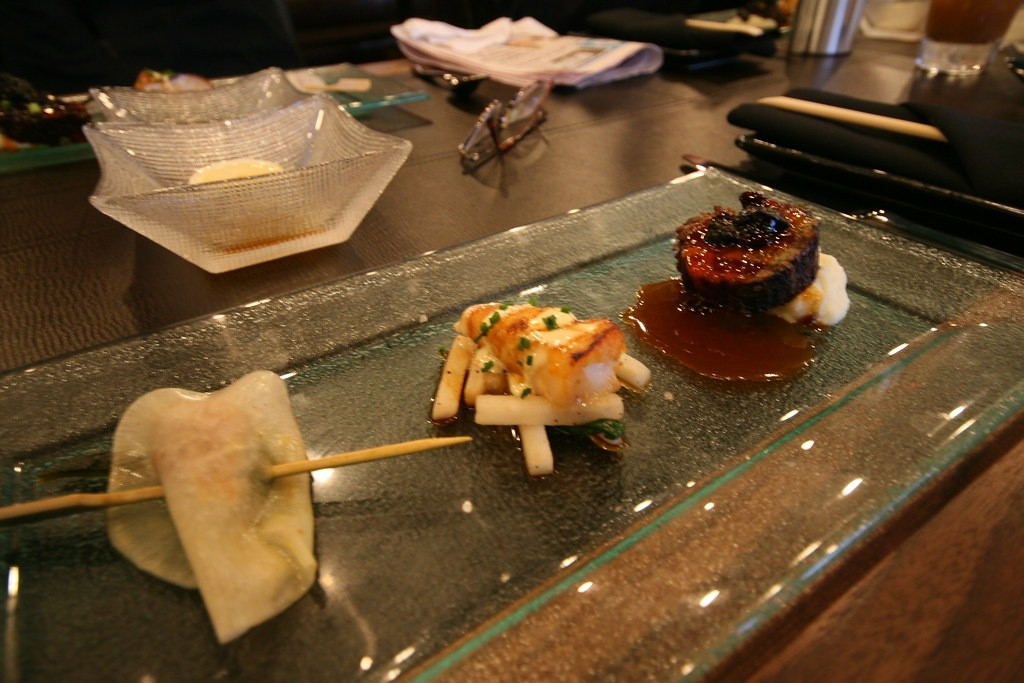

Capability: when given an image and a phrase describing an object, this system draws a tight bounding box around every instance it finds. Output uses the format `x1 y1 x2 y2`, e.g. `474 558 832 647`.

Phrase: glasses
456 77 552 174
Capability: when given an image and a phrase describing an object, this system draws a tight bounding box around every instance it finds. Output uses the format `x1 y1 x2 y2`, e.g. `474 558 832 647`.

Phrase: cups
789 0 864 56
916 0 1024 76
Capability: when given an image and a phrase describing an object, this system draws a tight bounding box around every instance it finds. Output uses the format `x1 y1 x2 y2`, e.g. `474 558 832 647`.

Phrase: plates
0 165 1024 683
0 61 431 176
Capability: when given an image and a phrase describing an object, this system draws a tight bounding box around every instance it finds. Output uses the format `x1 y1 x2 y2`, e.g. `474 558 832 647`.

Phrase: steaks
674 189 821 315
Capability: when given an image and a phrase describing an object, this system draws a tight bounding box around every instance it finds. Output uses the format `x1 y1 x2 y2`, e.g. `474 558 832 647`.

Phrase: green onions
436 296 572 398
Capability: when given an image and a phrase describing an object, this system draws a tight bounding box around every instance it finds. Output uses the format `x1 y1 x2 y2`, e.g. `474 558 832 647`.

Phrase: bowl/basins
80 67 413 274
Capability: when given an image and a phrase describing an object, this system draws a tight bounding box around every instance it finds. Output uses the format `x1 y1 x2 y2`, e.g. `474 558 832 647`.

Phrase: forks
414 62 490 90
